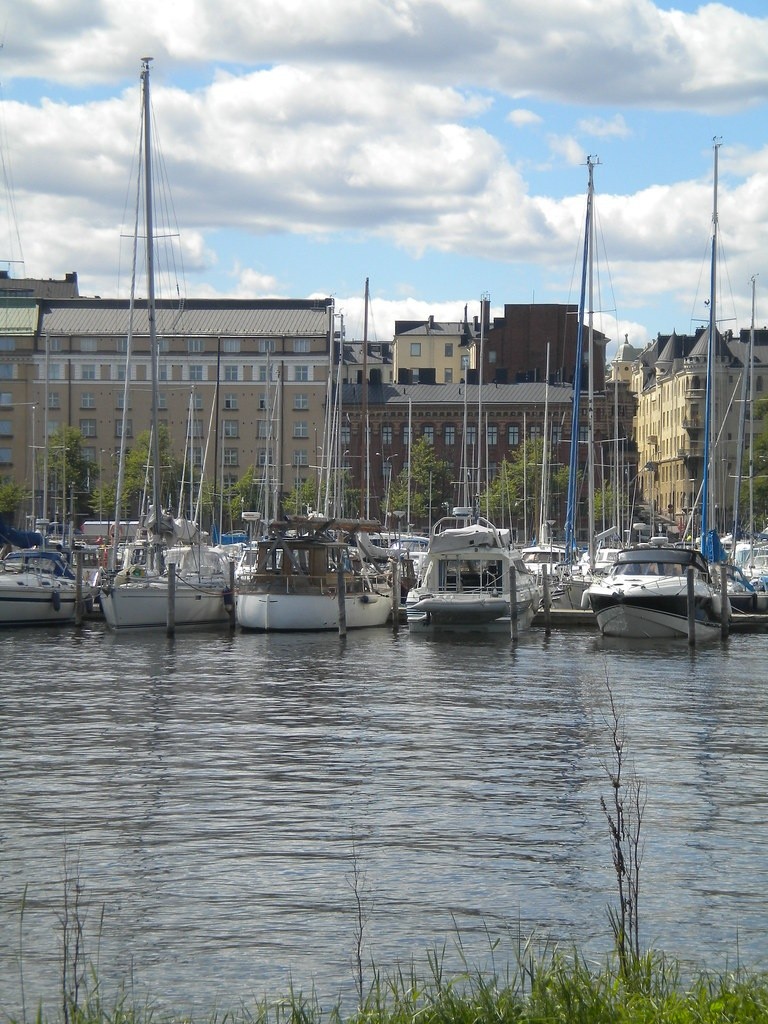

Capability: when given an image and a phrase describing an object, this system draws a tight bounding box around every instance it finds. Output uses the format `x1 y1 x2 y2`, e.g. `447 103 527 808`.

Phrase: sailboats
0 57 768 642
